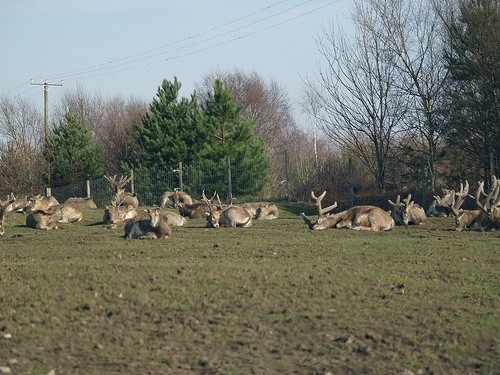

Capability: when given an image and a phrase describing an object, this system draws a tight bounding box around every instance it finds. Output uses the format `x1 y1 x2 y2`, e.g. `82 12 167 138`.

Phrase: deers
426 175 500 233
310 189 396 232
0 192 98 237
386 194 429 226
102 173 280 240
